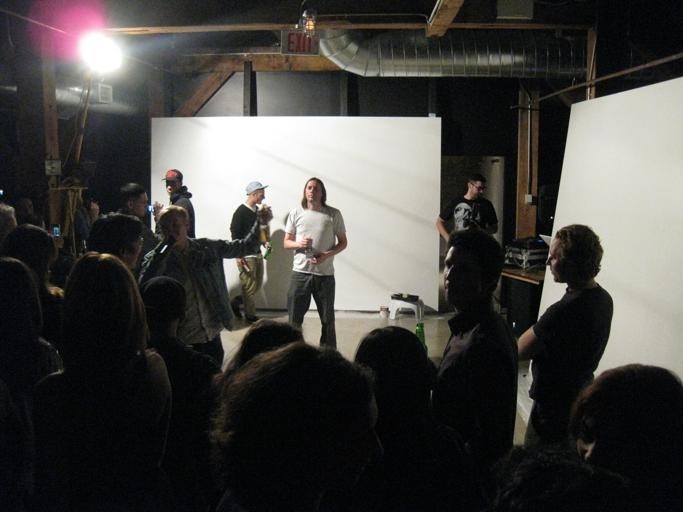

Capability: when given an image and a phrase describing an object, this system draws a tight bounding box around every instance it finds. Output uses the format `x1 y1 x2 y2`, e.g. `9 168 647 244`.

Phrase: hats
245 182 269 195
161 169 183 182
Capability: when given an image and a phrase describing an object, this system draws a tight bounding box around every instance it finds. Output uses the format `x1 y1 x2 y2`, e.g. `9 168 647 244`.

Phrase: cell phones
52 224 60 238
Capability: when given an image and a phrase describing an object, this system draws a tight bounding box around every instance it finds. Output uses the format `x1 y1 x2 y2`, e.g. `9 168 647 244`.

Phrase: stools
388 298 424 322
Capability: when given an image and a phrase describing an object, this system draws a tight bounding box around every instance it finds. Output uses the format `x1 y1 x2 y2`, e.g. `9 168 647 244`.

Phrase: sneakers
230 301 242 319
246 315 258 324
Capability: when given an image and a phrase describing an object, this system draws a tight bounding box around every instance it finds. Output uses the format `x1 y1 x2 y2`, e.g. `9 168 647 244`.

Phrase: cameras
147 205 156 212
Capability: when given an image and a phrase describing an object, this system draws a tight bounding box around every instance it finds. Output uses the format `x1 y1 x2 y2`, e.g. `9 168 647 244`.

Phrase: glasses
470 182 487 191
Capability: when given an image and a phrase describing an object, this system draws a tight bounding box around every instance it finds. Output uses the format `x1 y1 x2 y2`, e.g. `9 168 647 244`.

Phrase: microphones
160 232 176 255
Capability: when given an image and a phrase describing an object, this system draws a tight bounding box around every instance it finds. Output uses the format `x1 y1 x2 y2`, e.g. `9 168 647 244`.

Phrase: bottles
257 205 270 244
263 239 273 259
379 307 390 319
416 322 427 360
77 237 87 261
304 247 314 259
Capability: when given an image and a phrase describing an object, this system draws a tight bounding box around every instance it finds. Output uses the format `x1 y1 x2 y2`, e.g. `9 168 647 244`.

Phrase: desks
498 265 545 336
